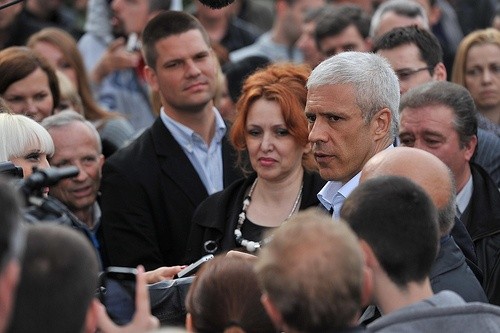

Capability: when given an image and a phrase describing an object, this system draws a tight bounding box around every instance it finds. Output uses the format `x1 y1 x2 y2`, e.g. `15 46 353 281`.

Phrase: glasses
395 66 433 80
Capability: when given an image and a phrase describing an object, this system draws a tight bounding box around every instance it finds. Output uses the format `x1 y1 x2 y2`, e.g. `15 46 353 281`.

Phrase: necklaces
235 178 303 252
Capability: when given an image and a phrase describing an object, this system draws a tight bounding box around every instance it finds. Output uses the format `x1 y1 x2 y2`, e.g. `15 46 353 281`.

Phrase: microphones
26 166 79 191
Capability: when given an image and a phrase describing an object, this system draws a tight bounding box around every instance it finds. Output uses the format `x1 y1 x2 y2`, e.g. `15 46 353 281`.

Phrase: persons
0 0 500 333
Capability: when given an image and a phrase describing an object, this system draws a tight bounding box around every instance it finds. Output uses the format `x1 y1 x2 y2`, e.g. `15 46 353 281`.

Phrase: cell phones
103 267 138 325
